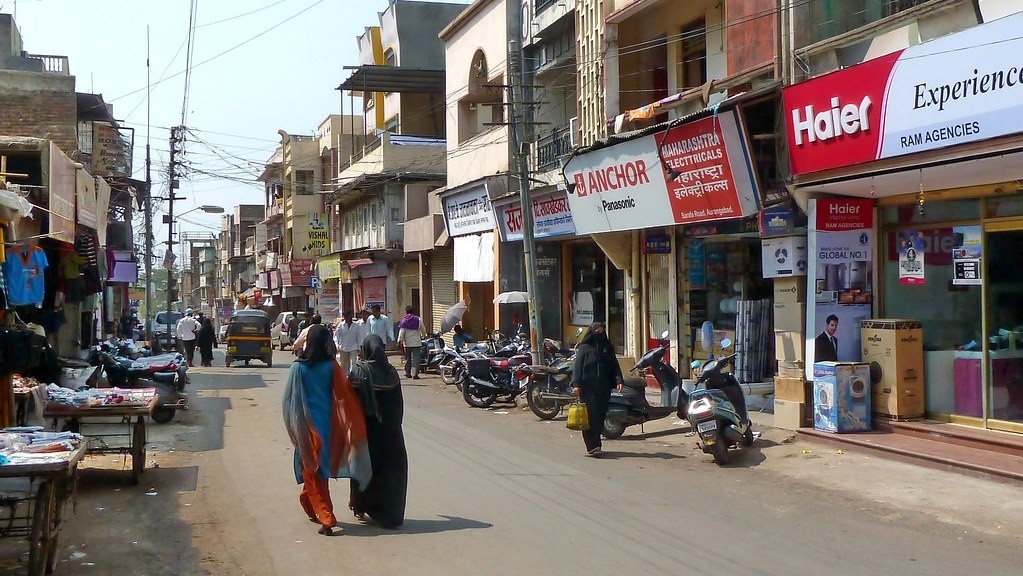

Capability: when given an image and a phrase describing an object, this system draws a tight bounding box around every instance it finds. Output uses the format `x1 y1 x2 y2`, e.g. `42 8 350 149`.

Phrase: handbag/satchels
566 395 590 430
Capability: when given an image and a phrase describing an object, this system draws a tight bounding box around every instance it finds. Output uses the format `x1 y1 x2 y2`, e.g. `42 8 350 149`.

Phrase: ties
830 337 834 350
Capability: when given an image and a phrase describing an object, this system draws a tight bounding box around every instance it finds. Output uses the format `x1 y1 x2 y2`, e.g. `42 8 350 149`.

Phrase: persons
570 322 624 456
282 324 372 538
105 308 218 368
512 314 526 338
348 334 408 528
816 314 840 362
287 305 426 379
452 326 471 351
900 234 915 251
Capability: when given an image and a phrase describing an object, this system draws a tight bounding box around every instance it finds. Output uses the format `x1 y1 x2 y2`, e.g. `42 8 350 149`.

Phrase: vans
155 311 181 345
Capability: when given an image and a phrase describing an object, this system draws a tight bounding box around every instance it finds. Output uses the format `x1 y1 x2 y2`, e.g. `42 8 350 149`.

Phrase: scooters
686 338 754 465
88 337 191 423
600 330 690 439
412 330 445 373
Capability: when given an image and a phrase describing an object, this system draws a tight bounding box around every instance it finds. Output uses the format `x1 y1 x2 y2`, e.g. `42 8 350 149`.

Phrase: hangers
7 235 44 256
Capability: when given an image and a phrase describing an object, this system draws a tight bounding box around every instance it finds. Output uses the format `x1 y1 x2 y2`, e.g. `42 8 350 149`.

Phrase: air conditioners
569 117 578 148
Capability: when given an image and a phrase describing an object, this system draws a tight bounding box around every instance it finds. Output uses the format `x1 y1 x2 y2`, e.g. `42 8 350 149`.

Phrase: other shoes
310 519 318 523
189 364 194 367
593 449 602 457
413 376 420 379
207 365 211 367
404 373 412 378
318 528 332 536
203 365 207 367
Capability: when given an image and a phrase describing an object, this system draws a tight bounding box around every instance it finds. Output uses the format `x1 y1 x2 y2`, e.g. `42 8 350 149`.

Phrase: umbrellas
494 291 529 304
441 300 468 334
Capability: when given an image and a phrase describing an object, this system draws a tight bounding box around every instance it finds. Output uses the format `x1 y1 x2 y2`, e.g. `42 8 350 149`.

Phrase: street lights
167 204 224 353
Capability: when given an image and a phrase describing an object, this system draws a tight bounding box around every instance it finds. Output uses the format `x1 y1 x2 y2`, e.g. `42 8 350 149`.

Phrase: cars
269 311 307 351
219 326 228 344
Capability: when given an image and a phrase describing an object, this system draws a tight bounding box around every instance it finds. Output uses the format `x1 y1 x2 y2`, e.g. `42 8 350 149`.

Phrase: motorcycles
509 327 583 419
434 321 562 408
224 310 273 368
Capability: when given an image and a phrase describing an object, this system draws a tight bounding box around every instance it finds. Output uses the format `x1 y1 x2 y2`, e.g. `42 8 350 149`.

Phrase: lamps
302 245 308 252
658 121 682 182
559 145 588 193
308 243 315 250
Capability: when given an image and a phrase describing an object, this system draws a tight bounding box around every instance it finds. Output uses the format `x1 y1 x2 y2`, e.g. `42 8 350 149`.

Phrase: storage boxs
692 329 735 381
470 358 490 375
762 233 810 432
814 361 870 434
860 317 925 421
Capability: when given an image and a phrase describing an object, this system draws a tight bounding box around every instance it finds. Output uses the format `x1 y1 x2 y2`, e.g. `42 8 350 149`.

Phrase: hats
185 308 193 316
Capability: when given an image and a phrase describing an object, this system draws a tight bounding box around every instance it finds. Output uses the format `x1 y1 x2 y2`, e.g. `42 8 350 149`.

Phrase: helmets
311 315 321 324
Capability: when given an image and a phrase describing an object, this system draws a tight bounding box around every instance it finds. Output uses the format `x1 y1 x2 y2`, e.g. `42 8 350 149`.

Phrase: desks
954 346 1023 419
48 386 159 486
12 381 47 427
0 426 88 540
